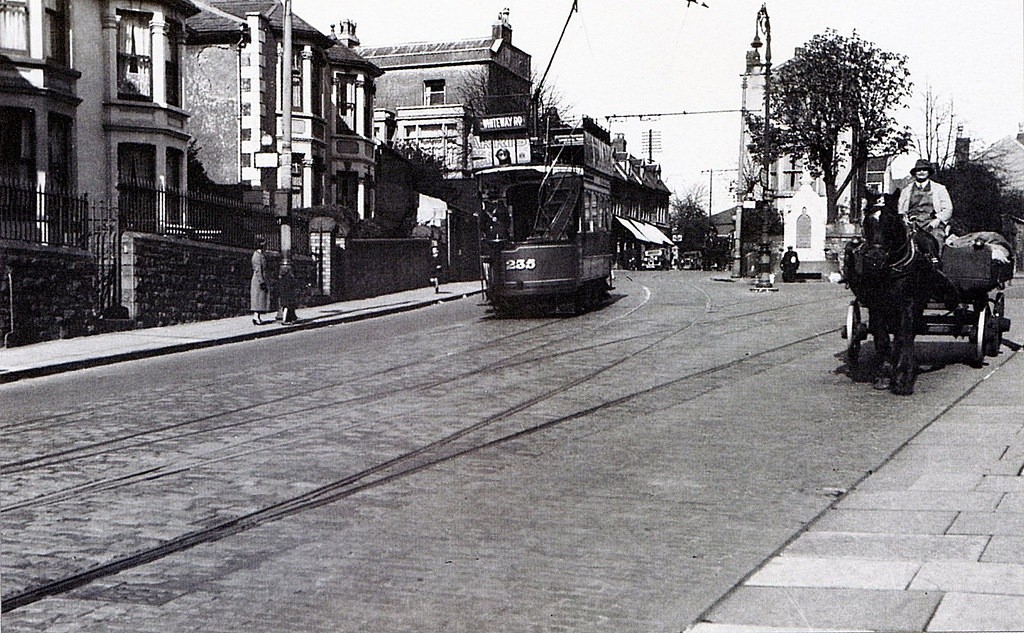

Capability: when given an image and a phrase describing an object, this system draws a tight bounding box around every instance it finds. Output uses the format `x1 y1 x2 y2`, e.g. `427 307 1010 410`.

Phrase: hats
910 158 934 177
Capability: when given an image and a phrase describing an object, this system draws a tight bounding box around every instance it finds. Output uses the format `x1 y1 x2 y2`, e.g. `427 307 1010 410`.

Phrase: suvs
641 250 662 270
681 251 702 270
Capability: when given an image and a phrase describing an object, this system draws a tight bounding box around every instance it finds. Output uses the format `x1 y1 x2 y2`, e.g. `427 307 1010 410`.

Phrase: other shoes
281 321 291 326
291 320 302 324
253 318 264 325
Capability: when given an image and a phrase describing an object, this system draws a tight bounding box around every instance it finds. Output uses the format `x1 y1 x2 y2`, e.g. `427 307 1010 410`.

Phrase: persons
426 242 442 295
249 236 298 326
891 158 954 266
779 245 800 284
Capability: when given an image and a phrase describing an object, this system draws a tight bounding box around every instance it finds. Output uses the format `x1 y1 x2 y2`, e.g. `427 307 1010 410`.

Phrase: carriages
842 179 1011 395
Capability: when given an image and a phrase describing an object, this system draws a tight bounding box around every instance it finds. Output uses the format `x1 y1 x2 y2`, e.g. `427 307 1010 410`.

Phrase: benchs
942 202 1014 281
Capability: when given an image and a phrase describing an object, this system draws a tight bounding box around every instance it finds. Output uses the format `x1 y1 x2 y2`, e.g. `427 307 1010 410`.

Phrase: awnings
615 216 674 246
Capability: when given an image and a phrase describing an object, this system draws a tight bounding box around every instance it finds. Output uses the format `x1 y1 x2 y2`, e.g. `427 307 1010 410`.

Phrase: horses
843 187 933 396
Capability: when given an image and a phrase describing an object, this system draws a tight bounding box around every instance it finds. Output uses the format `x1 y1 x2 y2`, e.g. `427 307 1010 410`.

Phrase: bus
475 165 613 313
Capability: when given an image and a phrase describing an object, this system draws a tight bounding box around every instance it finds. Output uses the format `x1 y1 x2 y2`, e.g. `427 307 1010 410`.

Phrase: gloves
261 283 266 290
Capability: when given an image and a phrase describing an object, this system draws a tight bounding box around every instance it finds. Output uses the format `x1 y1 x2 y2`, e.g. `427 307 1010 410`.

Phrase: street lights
748 2 779 293
